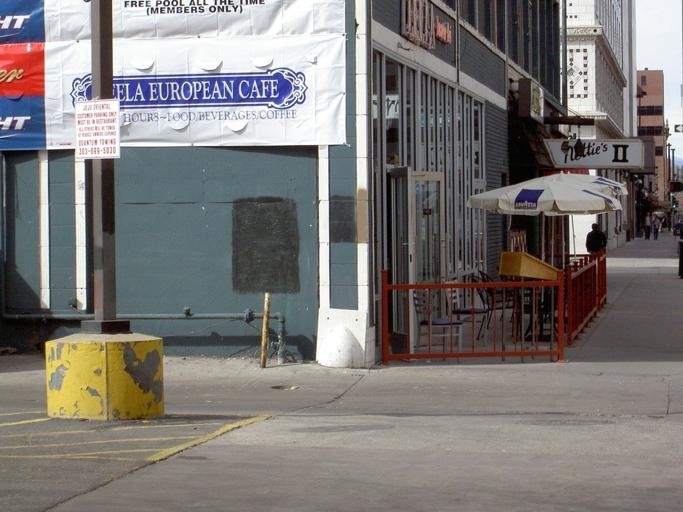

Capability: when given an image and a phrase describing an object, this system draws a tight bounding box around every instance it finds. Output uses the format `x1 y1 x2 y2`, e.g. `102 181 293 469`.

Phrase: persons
642 212 667 240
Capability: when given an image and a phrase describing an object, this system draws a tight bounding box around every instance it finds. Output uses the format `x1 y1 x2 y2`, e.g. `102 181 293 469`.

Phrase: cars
672 222 680 236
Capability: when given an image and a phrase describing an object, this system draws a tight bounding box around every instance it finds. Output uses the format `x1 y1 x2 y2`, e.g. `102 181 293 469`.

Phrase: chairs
412 271 514 363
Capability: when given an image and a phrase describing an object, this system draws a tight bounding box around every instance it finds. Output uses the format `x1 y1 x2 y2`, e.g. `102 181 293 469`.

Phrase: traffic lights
672 196 679 210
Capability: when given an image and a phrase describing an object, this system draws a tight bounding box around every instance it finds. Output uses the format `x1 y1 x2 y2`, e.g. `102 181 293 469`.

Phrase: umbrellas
464 170 629 301
